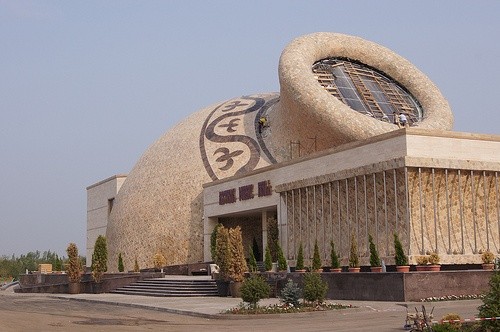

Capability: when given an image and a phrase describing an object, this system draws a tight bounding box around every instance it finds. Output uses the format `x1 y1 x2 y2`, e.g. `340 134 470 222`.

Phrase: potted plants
428 253 441 271
481 252 496 270
264 247 274 273
229 226 248 298
312 241 323 272
295 242 307 273
348 235 361 272
368 233 382 272
67 243 80 294
329 241 342 273
393 234 410 272
91 236 108 293
416 257 427 271
215 223 230 297
278 249 287 273
